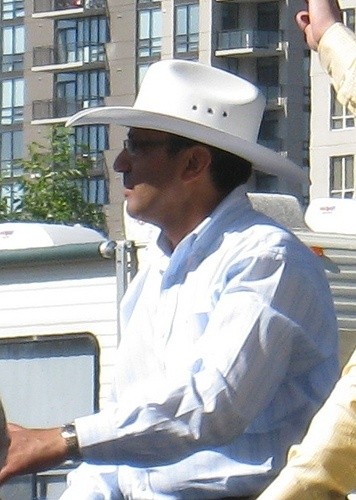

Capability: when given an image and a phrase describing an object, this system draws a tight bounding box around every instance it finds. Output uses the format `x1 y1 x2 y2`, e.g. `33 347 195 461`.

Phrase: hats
65 59 312 186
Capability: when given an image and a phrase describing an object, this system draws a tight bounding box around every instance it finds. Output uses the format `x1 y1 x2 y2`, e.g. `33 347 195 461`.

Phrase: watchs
60 424 80 462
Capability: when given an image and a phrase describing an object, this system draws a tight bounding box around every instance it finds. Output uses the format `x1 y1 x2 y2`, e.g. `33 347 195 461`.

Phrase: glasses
123 139 192 156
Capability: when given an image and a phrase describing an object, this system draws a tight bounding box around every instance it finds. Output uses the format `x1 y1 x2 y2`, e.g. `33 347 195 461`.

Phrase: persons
260 0 356 500
0 59 342 500
